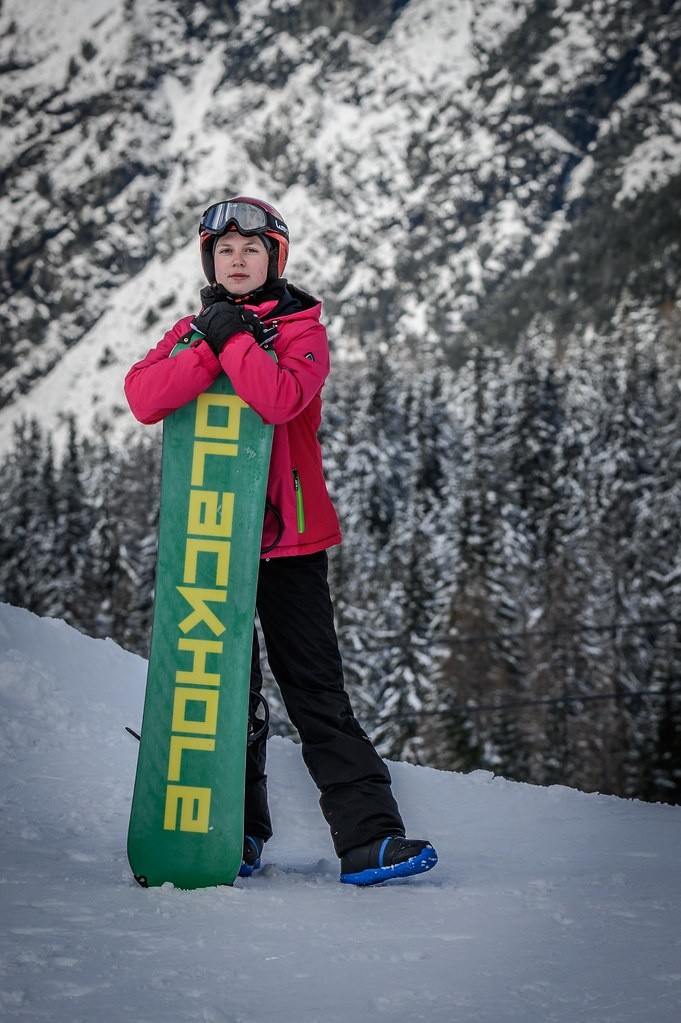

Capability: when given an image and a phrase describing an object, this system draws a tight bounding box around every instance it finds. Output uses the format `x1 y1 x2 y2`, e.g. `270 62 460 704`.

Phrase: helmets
199 197 289 284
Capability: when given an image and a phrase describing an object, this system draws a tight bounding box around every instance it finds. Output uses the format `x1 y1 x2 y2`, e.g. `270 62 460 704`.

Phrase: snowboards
127 332 280 897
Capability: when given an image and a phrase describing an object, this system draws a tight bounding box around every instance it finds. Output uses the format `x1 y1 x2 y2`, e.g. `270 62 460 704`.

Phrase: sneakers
340 834 438 886
238 834 264 877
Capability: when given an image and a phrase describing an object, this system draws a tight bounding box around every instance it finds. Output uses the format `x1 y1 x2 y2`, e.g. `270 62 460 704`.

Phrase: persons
121 197 439 891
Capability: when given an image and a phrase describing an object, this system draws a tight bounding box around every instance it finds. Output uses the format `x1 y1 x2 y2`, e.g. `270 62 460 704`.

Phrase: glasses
200 200 267 237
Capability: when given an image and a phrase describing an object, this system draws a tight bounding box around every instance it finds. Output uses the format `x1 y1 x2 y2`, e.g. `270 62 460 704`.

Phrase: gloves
203 307 278 357
190 301 242 355
